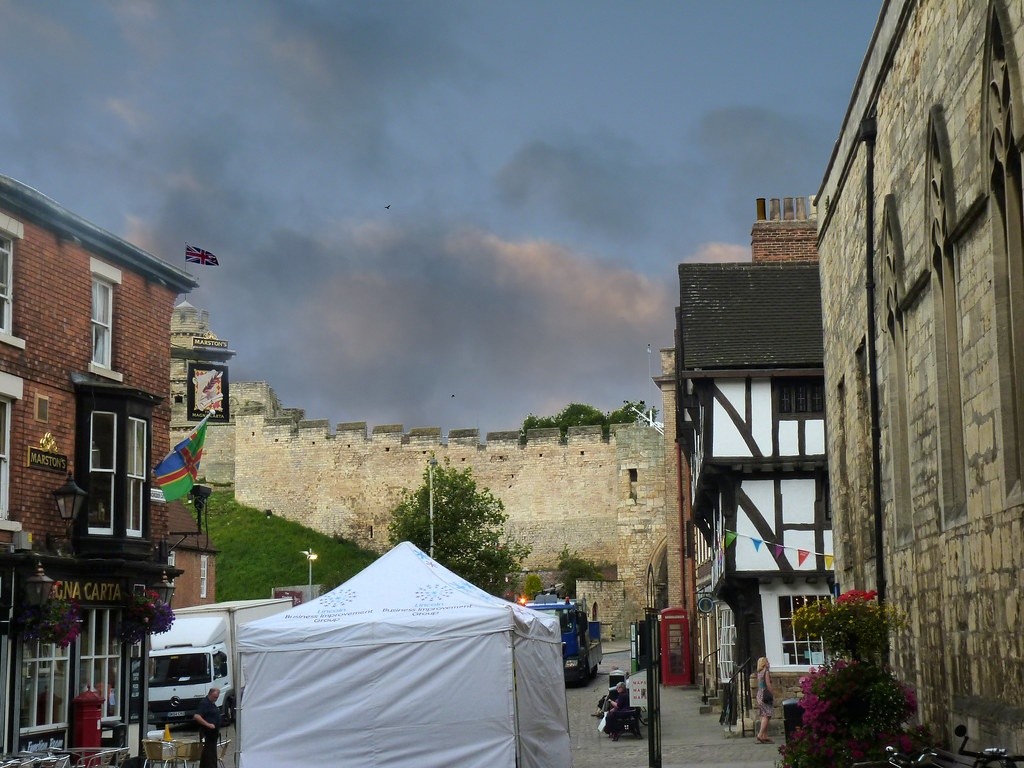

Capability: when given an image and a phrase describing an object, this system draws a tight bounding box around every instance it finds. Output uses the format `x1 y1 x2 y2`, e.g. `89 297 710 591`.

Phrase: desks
68 746 124 767
173 740 202 768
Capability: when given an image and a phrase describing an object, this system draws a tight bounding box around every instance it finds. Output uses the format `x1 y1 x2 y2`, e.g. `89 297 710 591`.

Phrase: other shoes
756 735 775 744
590 710 602 717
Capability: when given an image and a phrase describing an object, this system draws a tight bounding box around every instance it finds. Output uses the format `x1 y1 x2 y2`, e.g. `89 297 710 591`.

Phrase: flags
153 415 207 502
185 244 219 267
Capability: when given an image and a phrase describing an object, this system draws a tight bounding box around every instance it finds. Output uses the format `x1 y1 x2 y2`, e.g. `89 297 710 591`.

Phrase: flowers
791 587 912 655
116 587 172 645
10 599 84 649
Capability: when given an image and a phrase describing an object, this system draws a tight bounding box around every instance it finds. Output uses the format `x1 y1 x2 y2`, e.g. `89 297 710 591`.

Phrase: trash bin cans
100 720 127 767
782 699 806 747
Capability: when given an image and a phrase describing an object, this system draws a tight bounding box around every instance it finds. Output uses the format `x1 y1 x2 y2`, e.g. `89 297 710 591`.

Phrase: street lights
299 547 318 585
429 457 440 559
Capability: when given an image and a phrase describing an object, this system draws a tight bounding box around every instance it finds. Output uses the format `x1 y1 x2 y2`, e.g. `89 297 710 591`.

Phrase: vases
834 631 859 650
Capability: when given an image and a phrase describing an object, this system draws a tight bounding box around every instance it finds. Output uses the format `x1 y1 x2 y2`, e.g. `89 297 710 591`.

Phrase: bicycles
852 722 1024 768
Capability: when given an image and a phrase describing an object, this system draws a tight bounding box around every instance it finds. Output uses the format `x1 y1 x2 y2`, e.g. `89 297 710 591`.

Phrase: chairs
0 735 240 768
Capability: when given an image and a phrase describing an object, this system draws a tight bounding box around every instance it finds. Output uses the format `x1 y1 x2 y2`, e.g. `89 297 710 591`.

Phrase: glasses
616 687 621 689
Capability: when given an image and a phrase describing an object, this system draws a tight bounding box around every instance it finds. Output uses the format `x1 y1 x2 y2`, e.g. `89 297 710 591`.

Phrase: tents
235 541 574 768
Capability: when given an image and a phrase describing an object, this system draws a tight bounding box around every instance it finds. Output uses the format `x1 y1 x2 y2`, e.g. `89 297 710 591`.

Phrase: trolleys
141 731 231 768
0 747 130 768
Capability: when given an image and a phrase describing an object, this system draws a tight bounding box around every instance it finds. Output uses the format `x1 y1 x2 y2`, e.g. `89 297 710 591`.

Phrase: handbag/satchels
761 688 773 706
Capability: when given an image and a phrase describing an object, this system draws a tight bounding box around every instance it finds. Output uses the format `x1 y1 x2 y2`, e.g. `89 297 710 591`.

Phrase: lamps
45 469 87 550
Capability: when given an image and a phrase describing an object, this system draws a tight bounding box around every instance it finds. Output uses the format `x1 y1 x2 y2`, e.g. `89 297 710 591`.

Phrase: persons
754 658 777 743
604 680 630 738
195 656 222 678
194 688 221 768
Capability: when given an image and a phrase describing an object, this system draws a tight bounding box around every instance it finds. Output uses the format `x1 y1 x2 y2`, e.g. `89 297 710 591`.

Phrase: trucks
524 593 602 688
147 597 294 731
271 584 321 607
